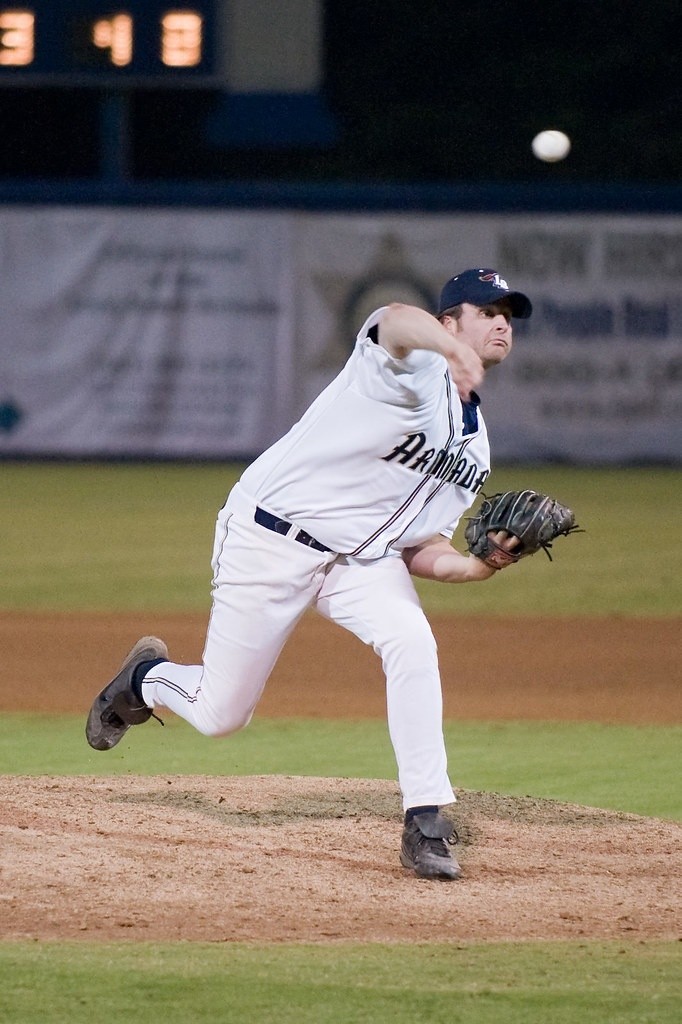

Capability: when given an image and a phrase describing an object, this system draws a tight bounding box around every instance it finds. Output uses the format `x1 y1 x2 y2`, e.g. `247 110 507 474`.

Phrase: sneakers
400 814 465 881
85 635 171 753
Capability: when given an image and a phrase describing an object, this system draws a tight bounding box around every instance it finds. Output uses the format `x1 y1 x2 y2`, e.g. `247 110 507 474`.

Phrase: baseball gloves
463 488 577 571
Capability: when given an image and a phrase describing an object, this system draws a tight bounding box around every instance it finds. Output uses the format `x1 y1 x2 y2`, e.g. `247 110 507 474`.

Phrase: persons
83 266 535 883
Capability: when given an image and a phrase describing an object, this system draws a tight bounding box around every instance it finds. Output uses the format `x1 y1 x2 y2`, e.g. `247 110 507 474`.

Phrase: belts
254 508 332 556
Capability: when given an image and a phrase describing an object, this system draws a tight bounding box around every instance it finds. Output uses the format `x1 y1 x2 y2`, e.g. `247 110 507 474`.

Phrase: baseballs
531 130 572 164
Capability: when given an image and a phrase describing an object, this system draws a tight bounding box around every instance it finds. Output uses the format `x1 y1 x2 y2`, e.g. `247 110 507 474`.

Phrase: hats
438 266 532 319
